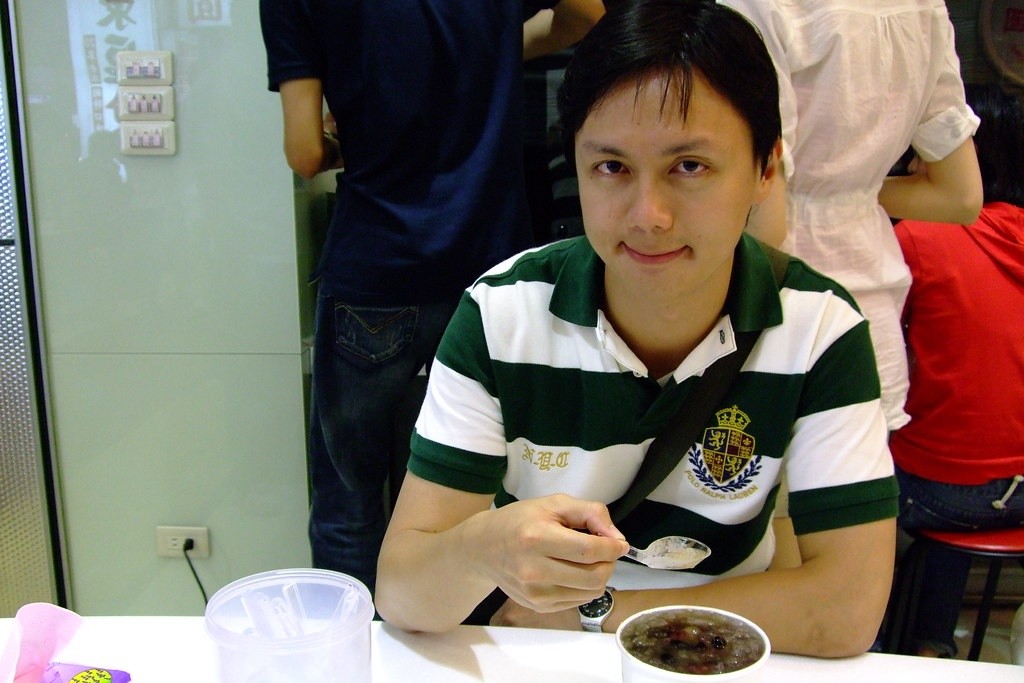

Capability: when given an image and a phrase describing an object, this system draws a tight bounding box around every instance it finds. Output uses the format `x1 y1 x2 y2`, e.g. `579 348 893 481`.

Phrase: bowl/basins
205 568 374 683
617 606 771 683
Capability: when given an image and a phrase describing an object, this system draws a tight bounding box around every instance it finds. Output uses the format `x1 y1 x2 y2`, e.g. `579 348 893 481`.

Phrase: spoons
625 537 711 570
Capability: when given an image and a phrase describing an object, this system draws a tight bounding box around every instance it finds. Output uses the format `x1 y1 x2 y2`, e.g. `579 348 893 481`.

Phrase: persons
258 0 1024 659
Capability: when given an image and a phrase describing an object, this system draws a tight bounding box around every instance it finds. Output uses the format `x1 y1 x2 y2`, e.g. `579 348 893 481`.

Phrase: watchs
577 587 615 633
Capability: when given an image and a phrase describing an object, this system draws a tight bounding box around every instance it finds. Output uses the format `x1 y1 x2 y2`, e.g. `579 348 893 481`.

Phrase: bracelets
323 131 342 160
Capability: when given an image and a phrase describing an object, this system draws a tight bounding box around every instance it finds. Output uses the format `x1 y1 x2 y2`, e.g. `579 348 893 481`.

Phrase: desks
1 619 1022 682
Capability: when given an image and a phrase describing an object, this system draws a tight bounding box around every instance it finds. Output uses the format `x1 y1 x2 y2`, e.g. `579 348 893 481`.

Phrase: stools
896 521 1024 663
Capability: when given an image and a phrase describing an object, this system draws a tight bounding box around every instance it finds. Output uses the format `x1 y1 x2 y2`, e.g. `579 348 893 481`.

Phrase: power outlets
156 524 209 558
